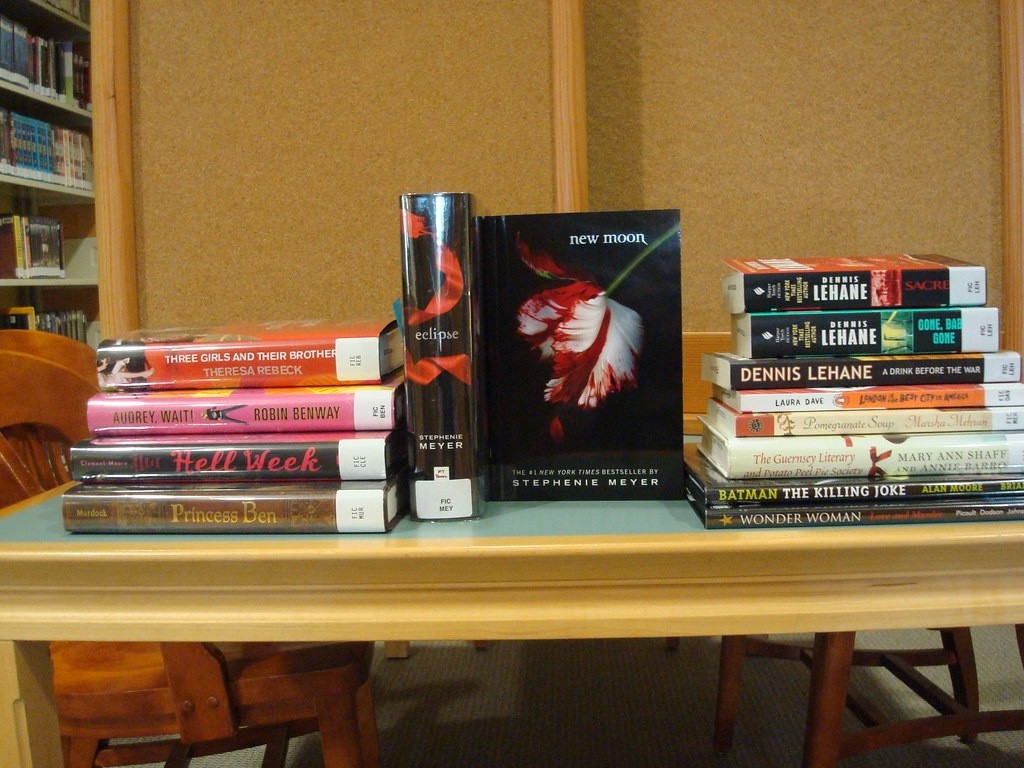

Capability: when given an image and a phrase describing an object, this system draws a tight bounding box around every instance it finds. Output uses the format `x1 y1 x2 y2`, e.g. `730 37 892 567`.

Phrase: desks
0 481 1024 768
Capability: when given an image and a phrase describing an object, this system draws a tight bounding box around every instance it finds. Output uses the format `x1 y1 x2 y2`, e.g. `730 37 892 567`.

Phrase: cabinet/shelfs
0 0 100 355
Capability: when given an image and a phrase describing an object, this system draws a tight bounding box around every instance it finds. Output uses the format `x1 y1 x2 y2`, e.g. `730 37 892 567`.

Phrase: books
41 0 90 24
464 207 687 503
684 254 1023 531
61 315 408 534
0 107 94 193
0 211 66 281
0 305 88 346
0 13 92 113
398 191 481 521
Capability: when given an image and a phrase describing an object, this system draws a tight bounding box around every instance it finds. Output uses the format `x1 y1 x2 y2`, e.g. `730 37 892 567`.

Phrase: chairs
714 611 1024 768
681 330 737 445
0 329 376 768
